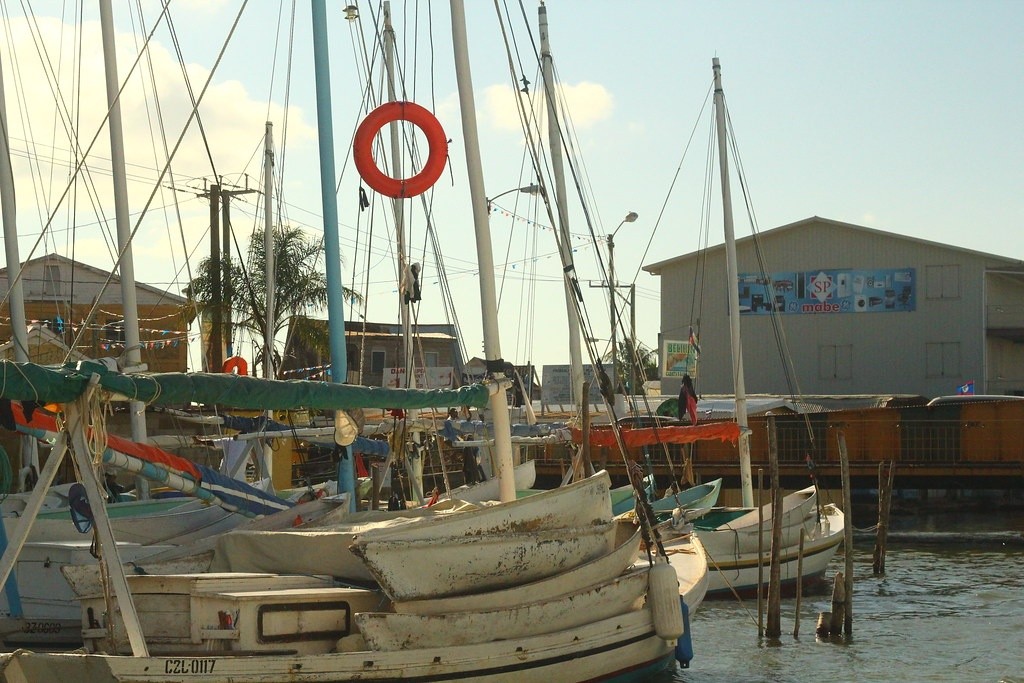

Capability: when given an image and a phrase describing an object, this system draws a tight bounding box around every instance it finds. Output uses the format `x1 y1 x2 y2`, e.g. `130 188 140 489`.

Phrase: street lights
606 212 639 393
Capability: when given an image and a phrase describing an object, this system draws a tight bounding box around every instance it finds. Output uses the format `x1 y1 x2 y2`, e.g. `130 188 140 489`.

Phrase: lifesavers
223 355 250 374
351 99 449 198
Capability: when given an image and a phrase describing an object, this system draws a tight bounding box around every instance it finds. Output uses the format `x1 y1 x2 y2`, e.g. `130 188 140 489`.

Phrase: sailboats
0 2 855 683
